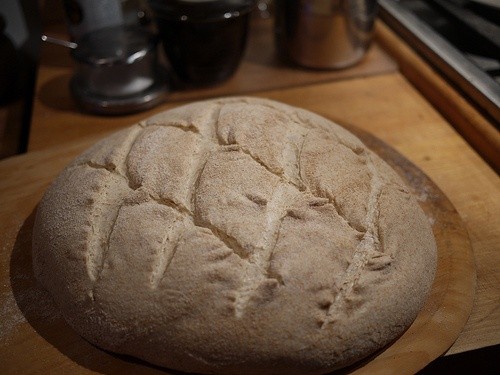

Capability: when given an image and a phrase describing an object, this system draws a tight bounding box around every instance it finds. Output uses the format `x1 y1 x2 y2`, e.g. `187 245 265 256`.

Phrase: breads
33 96 436 375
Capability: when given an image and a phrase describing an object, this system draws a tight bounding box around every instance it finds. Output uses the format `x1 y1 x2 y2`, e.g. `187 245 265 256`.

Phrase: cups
70 24 173 115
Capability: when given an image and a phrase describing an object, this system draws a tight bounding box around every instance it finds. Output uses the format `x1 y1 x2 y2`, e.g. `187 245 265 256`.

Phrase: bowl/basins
146 3 248 91
273 1 378 70
